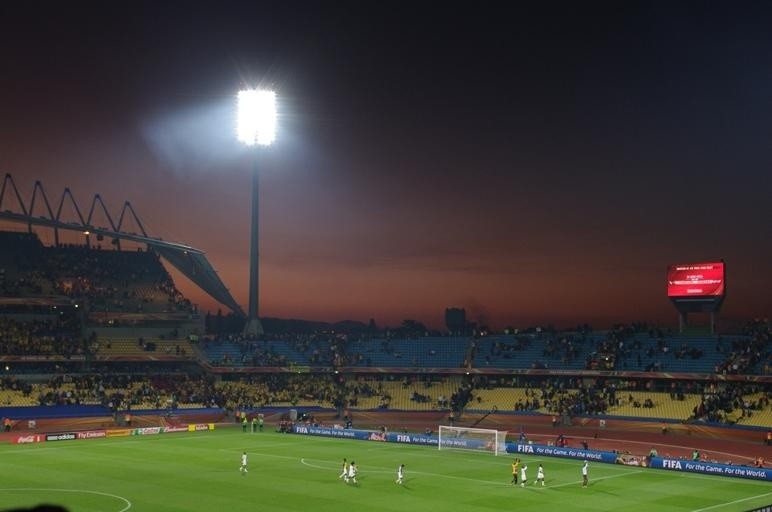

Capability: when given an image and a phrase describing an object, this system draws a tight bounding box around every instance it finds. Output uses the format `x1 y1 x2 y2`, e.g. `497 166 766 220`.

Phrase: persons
238 449 250 474
520 465 527 488
581 460 588 489
347 462 358 486
396 464 405 486
339 458 349 484
198 317 772 469
0 242 198 433
534 463 546 487
510 460 518 485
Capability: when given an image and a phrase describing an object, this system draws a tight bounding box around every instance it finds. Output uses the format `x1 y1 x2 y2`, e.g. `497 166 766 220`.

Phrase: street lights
235 87 278 338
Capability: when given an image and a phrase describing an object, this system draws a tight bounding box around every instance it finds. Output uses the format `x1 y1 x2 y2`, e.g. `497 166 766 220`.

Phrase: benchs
1 334 772 428
